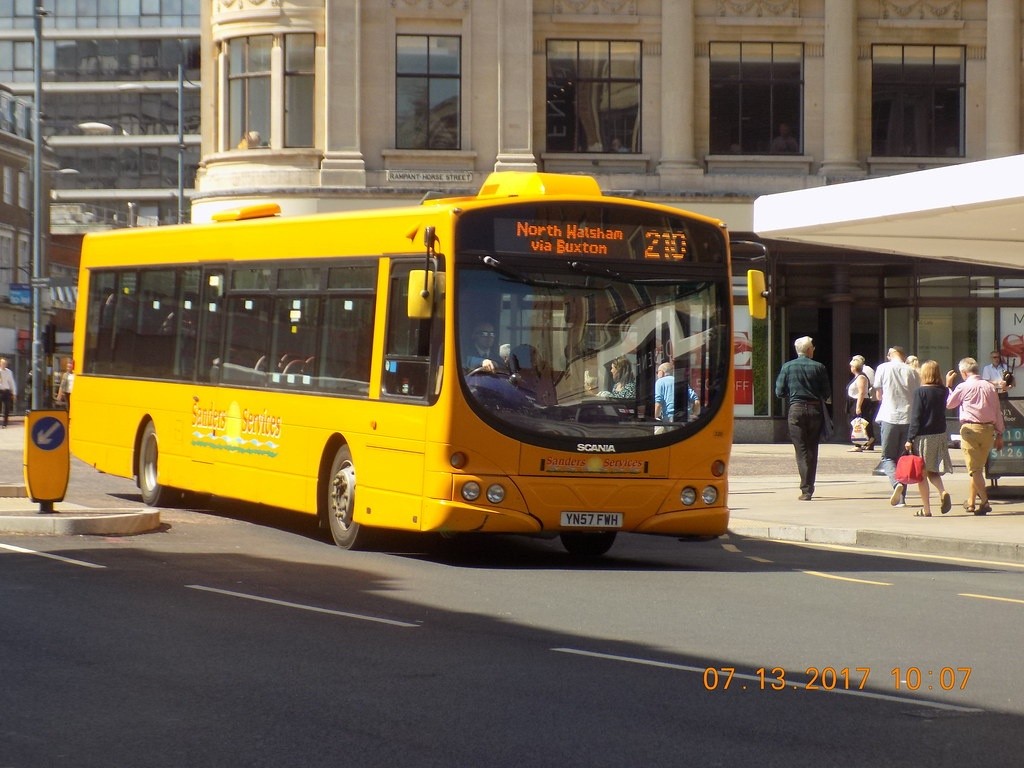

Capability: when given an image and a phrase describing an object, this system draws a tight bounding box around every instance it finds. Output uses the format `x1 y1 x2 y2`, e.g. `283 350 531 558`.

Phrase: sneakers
846 447 863 452
861 437 874 449
799 493 812 500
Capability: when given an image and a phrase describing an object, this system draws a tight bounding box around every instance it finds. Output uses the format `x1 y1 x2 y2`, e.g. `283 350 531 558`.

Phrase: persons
461 320 509 379
23 368 33 410
654 361 700 435
56 362 74 413
844 345 1016 517
610 355 636 414
0 357 18 428
774 335 830 501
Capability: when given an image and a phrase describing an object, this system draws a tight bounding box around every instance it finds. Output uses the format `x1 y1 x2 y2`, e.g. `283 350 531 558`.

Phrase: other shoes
913 508 932 517
940 491 952 514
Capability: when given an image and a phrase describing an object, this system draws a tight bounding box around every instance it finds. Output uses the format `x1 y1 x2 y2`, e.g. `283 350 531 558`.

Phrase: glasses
992 357 1001 359
477 331 496 338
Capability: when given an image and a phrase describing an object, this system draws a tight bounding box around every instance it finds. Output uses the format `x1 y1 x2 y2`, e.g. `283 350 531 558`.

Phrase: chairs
96 294 371 398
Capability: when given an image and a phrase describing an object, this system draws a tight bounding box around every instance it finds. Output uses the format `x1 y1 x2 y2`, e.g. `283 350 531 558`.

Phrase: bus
67 170 770 557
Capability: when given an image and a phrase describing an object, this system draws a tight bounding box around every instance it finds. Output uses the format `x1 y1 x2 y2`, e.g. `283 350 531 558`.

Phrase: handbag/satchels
820 398 834 444
850 415 870 445
894 449 924 484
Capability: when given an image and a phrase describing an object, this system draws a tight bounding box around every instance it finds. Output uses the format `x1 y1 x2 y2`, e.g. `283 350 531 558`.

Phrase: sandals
963 499 975 512
974 499 992 516
